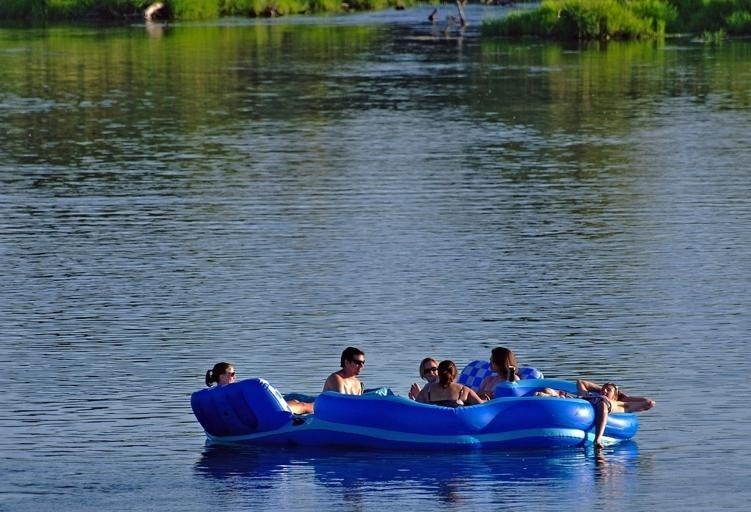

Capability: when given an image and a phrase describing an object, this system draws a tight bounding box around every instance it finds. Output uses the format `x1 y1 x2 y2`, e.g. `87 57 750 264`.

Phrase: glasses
424 367 437 374
351 358 366 365
606 382 619 394
230 371 236 378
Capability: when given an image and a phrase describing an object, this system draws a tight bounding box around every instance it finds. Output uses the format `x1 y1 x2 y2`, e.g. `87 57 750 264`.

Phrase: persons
415 359 490 408
205 361 315 415
534 379 657 448
479 346 521 392
408 357 440 400
322 346 366 396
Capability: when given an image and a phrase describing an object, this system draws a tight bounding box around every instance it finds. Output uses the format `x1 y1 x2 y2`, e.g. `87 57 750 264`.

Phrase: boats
189 359 639 457
